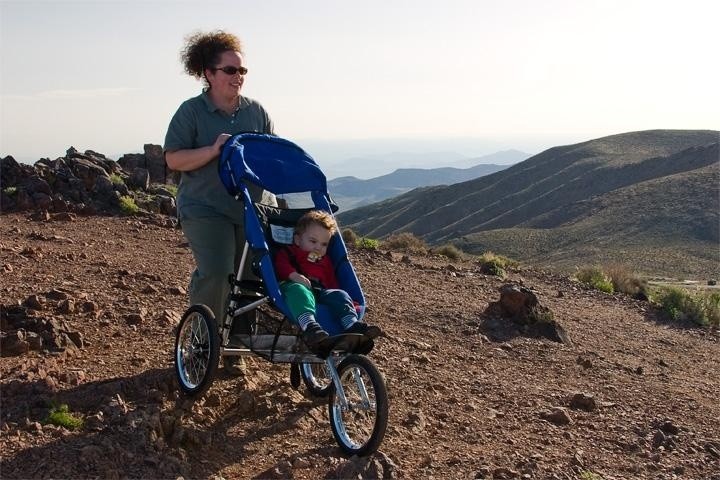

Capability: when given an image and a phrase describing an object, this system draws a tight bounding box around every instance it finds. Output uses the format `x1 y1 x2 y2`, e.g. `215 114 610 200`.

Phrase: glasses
215 65 247 75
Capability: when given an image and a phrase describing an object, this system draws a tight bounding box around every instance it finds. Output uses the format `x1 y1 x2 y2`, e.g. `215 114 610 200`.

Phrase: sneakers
305 322 327 344
354 321 380 340
193 342 207 369
223 356 246 375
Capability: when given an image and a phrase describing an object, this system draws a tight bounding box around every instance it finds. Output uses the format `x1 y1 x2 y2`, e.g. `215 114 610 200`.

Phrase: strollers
176 130 390 457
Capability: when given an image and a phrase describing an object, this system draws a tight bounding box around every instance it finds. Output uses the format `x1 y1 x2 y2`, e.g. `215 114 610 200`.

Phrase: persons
162 30 279 377
275 209 381 349
